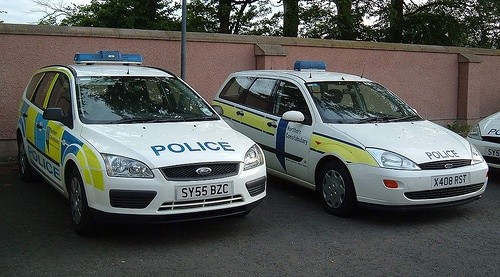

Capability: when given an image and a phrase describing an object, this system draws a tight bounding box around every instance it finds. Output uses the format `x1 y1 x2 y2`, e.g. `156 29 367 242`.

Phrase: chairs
127 80 151 107
325 89 354 121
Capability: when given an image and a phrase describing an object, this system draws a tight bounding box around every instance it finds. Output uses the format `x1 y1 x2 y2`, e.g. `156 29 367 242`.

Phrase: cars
466 108 500 173
16 49 269 236
212 60 489 218
